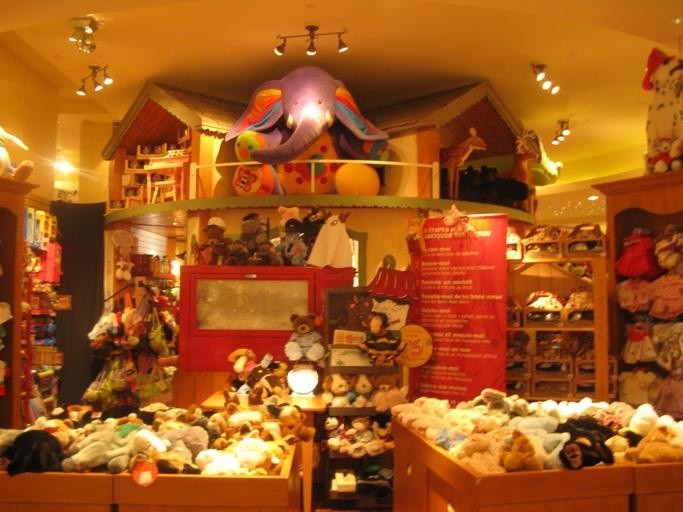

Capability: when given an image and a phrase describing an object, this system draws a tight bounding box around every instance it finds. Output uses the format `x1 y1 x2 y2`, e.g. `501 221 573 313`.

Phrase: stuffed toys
650 135 683 172
1 125 35 181
0 210 683 491
223 65 391 197
640 45 683 172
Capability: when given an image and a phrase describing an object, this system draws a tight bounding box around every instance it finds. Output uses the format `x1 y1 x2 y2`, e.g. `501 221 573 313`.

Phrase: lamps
76 65 113 96
272 24 348 57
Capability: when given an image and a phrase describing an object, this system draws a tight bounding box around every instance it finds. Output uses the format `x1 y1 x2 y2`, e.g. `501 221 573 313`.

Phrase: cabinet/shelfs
591 170 682 420
313 285 403 511
0 175 33 432
506 223 608 402
